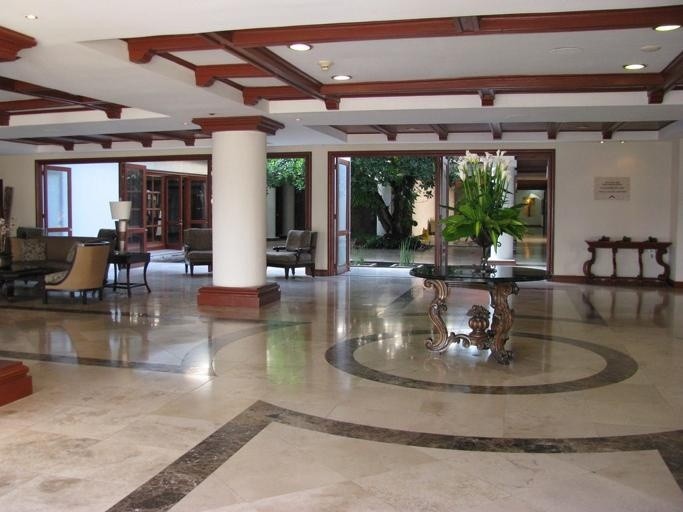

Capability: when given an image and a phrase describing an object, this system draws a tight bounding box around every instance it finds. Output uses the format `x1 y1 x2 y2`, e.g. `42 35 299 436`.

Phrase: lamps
109 201 132 254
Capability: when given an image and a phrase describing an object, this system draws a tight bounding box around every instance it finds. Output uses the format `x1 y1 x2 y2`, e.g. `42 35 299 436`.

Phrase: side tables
104 251 151 298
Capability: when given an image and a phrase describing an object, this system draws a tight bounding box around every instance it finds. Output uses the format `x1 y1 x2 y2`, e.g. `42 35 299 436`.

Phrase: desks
409 265 551 366
583 236 672 287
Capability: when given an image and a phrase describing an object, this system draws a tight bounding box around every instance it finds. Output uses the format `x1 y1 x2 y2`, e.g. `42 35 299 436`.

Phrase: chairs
184 228 213 276
266 230 318 280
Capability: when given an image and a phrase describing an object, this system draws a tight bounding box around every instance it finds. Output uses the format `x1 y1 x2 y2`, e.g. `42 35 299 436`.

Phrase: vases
470 234 501 273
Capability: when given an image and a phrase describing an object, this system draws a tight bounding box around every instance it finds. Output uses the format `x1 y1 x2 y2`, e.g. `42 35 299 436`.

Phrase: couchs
5 237 111 304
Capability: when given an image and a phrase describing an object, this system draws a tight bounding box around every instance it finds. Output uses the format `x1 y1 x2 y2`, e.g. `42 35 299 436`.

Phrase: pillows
9 237 85 263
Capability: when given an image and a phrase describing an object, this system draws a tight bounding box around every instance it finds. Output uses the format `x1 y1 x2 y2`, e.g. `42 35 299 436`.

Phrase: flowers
437 150 529 253
